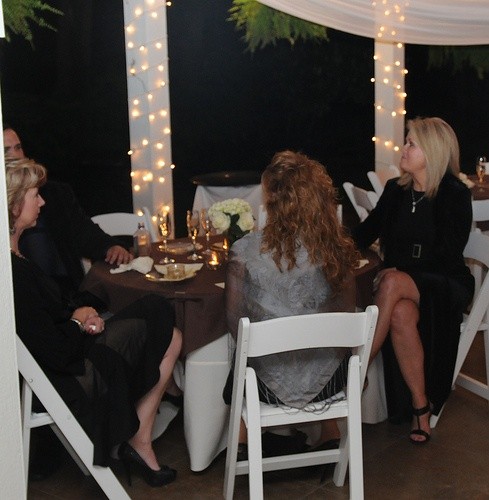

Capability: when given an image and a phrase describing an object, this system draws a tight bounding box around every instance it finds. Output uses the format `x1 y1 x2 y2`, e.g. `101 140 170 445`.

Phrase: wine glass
200 208 214 255
157 210 171 252
476 157 486 187
187 210 203 260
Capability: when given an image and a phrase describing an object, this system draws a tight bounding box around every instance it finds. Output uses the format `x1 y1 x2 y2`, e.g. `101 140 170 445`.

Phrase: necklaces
9 248 27 260
410 189 427 213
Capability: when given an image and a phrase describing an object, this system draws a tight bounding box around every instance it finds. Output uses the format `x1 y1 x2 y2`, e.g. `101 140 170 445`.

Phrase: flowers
208 198 255 233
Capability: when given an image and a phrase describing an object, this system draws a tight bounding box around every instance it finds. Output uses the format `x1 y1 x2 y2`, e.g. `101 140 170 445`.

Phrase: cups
167 263 185 279
205 251 222 270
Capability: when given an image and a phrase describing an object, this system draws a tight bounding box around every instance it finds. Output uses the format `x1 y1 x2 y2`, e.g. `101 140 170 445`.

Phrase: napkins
154 262 204 274
110 256 154 274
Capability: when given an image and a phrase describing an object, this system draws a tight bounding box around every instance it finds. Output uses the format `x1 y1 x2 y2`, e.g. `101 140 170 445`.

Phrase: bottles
132 222 152 259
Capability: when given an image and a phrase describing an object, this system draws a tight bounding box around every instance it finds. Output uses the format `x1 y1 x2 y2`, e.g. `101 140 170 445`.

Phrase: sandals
409 400 432 445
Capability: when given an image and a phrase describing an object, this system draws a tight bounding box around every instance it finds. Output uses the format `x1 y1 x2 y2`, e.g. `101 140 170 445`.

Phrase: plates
144 270 195 282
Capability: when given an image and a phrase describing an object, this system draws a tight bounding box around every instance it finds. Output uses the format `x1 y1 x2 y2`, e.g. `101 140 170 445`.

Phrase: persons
348 118 473 446
218 152 360 485
2 125 183 487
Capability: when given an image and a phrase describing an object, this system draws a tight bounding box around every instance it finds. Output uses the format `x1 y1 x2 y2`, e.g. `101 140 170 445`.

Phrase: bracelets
69 317 85 329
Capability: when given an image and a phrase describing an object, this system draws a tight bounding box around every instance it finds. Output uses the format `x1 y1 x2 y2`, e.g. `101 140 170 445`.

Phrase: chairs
0 171 489 500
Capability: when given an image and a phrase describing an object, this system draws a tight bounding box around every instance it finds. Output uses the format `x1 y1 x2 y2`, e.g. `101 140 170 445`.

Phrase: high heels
300 438 340 484
237 443 267 461
157 392 184 415
118 441 178 487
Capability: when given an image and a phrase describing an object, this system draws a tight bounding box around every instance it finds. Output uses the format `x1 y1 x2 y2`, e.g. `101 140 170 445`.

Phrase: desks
81 238 382 357
